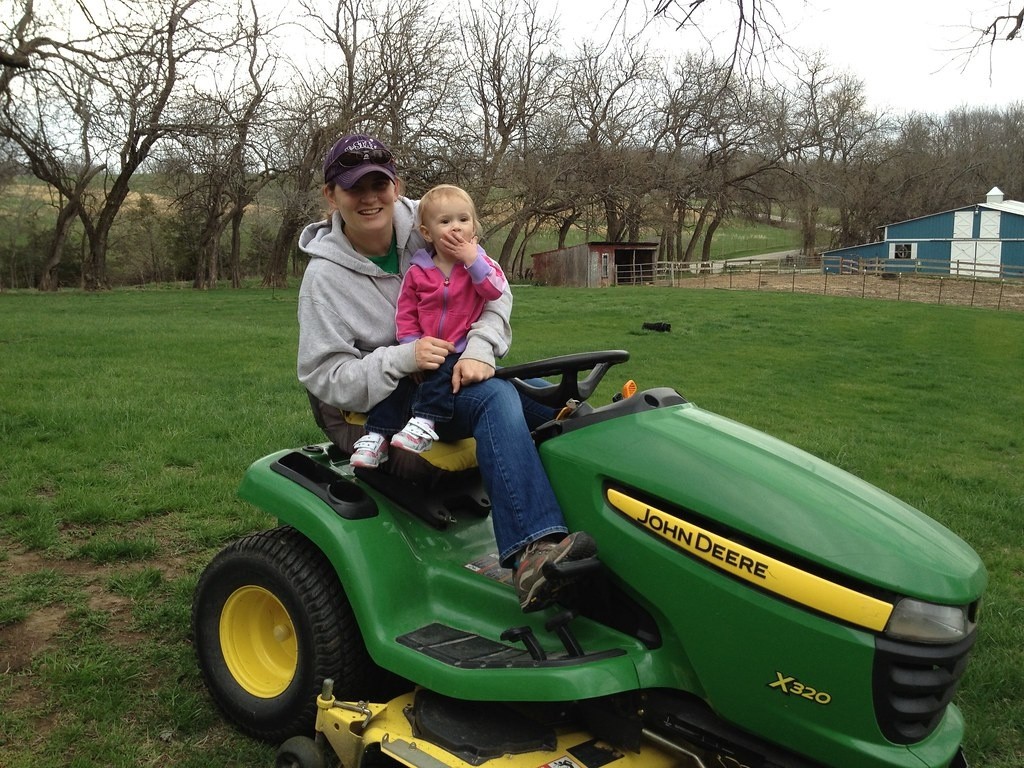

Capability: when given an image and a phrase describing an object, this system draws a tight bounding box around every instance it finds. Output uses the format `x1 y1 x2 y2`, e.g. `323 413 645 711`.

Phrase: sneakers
390 417 440 454
350 432 390 468
513 531 597 615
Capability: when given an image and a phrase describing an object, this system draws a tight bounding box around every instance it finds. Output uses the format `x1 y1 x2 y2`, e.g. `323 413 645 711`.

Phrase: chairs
307 388 497 531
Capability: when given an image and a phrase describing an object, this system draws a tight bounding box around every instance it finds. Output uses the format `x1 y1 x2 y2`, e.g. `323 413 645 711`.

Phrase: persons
350 186 505 470
296 131 600 612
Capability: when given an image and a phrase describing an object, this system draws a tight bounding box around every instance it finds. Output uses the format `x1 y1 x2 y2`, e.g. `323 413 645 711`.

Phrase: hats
324 132 396 190
